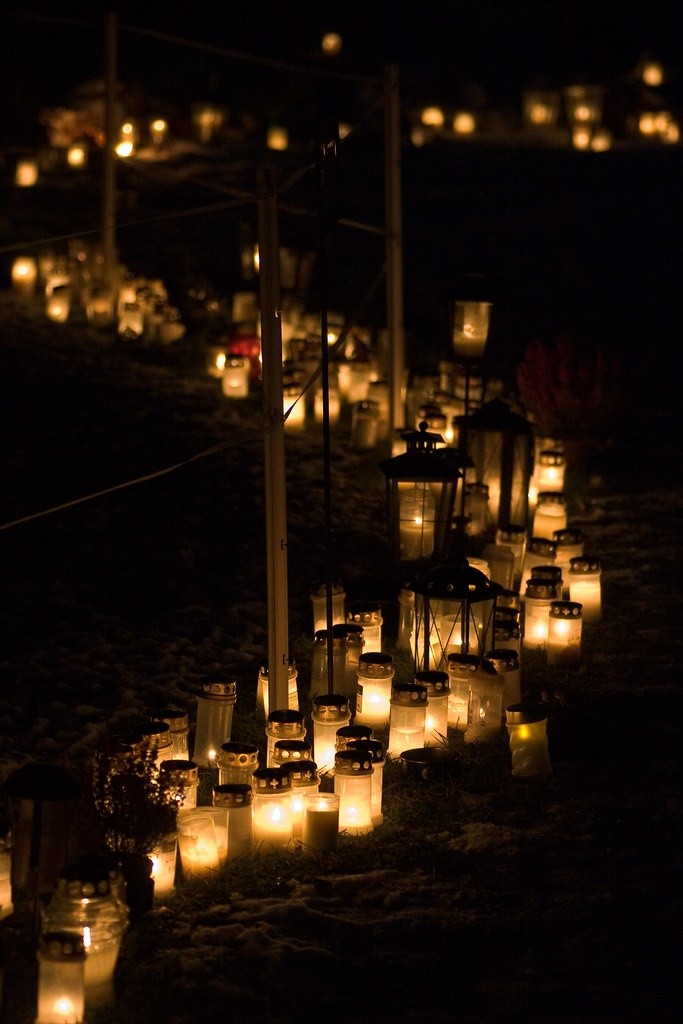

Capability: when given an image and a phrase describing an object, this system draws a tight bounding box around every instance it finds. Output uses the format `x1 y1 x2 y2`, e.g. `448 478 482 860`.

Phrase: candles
301 792 340 854
176 816 221 881
147 828 179 900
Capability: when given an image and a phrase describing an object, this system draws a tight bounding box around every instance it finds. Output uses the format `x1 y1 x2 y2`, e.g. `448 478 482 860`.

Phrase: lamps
344 739 386 828
279 761 321 842
150 710 190 761
504 699 553 781
192 623 582 771
0 762 83 900
156 759 200 810
332 749 374 838
212 784 256 860
0 826 14 920
136 721 174 768
39 241 642 623
36 931 86 1024
40 853 130 1008
215 742 260 785
251 768 293 851
